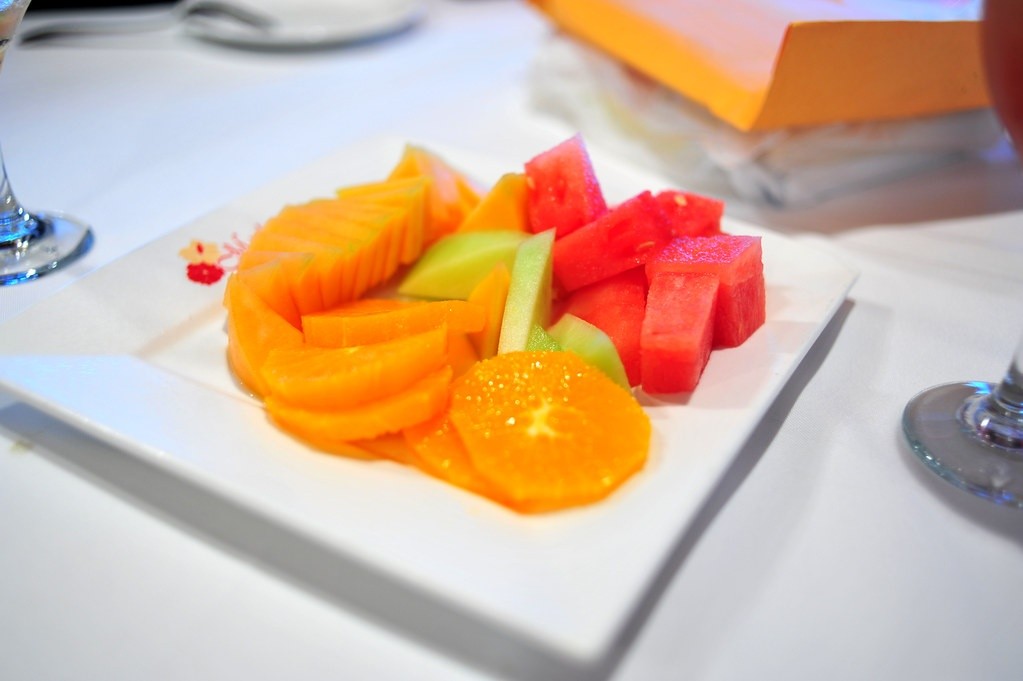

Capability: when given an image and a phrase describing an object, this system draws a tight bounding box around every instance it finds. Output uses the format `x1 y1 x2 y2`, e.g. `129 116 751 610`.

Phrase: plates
0 121 860 663
181 1 423 46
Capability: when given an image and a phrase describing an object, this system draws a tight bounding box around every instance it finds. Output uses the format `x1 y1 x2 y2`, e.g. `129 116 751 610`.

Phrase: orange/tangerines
221 277 654 511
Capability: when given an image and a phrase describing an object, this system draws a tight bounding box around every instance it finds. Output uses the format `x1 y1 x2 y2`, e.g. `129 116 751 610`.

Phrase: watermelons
526 133 766 396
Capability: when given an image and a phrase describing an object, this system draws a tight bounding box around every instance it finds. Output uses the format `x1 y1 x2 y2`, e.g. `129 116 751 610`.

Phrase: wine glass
0 1 94 286
900 329 1022 512
